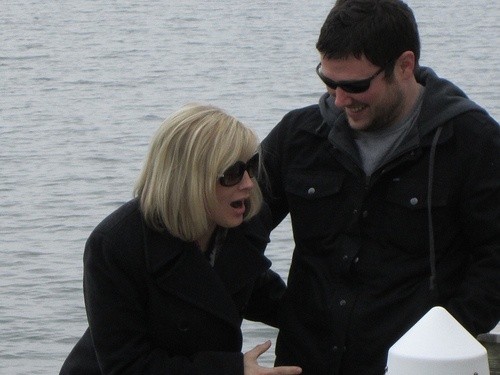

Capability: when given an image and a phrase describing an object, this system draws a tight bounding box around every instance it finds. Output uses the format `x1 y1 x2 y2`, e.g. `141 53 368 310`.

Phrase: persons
59 103 303 375
245 0 499 375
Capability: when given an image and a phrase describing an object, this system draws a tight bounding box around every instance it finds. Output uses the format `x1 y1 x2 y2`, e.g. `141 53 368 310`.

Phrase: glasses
219 152 259 186
316 62 394 93
